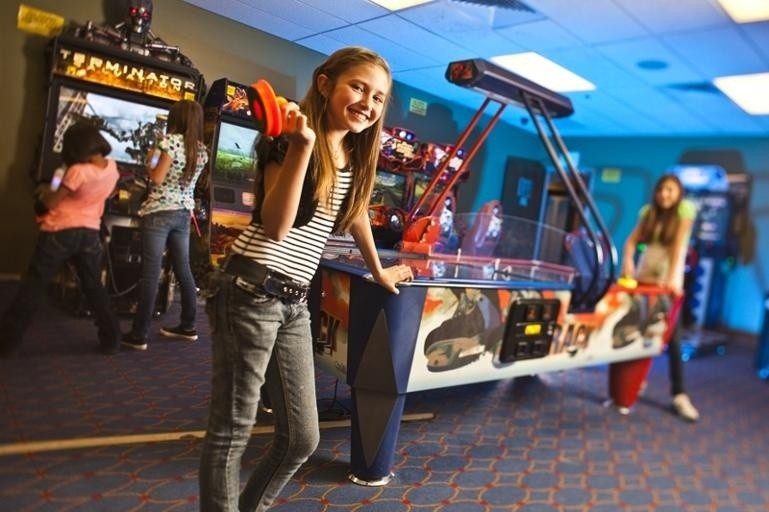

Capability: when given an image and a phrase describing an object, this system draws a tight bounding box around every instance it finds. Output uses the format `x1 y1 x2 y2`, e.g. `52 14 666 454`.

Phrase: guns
71 111 121 142
124 114 169 165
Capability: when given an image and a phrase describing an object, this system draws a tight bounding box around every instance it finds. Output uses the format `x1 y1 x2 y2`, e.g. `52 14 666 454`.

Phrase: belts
220 253 310 304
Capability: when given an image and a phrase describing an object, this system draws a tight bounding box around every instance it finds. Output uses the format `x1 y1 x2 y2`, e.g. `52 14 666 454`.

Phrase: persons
622 176 700 421
1 116 119 359
199 47 415 511
120 99 209 350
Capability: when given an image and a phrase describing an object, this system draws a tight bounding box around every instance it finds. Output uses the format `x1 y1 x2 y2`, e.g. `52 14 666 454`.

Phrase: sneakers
673 393 698 421
116 334 148 350
160 325 198 341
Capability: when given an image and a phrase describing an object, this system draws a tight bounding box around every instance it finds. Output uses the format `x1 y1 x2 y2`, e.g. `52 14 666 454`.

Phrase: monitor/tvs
375 173 406 208
41 80 170 178
210 117 259 188
416 182 463 211
